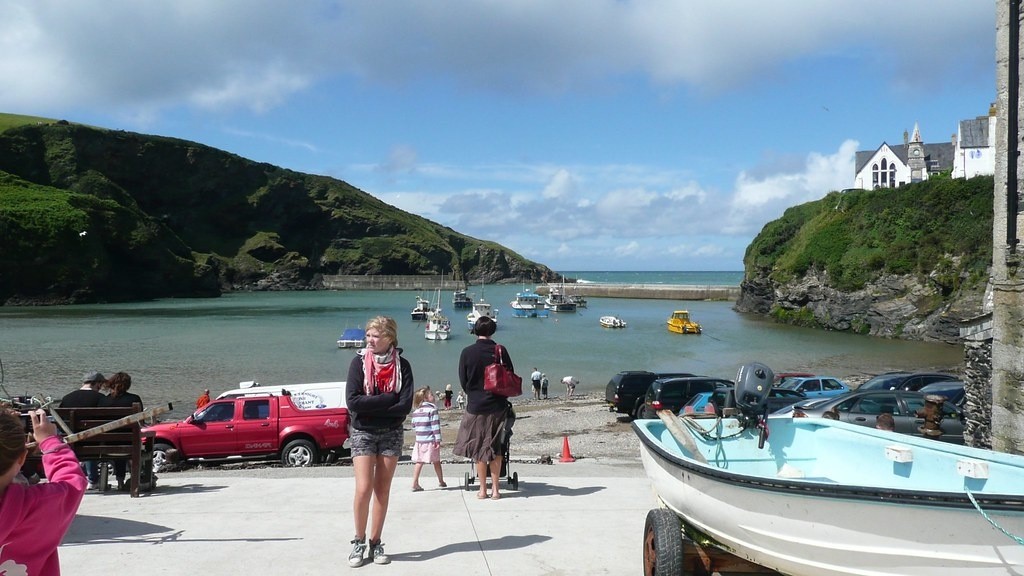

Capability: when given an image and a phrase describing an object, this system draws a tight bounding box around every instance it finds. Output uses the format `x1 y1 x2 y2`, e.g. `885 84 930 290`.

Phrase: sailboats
423 269 451 341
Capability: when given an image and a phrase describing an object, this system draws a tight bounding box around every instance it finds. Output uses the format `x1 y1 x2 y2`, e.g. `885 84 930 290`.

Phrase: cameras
17 413 41 433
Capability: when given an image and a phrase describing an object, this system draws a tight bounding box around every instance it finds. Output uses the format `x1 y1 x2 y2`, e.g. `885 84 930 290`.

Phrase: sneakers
368 538 389 564
349 534 367 568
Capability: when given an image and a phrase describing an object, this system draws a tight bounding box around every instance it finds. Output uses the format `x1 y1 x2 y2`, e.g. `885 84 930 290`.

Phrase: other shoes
87 482 98 490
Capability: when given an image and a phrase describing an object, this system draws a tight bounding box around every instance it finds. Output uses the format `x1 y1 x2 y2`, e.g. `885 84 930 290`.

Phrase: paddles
654 409 710 466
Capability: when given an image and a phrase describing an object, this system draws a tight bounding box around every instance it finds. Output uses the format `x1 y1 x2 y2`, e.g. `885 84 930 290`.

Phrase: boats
666 310 703 335
466 277 500 331
337 325 367 349
600 315 627 328
632 359 1023 576
451 287 475 308
410 295 435 320
509 272 588 318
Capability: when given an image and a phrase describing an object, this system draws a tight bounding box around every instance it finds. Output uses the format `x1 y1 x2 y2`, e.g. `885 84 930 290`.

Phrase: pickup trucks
141 388 351 474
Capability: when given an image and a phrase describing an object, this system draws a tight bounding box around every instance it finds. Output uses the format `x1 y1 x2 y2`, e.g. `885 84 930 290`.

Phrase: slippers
491 493 501 500
476 492 487 499
440 482 447 487
412 487 424 492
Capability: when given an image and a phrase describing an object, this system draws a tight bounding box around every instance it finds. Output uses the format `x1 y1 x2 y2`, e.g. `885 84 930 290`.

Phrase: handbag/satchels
483 343 522 397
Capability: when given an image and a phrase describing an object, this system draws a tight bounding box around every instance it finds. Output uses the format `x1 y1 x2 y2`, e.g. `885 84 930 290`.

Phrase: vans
198 380 352 464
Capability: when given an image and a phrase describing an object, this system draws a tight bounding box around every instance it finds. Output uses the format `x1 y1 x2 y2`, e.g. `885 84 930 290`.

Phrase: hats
83 371 108 383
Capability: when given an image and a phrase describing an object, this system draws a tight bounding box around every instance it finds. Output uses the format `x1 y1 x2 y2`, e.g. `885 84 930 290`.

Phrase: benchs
15 401 156 499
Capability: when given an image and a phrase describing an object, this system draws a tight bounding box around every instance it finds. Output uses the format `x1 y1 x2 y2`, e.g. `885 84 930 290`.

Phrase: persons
101 372 143 491
531 367 542 400
541 374 548 399
197 389 210 409
452 316 514 500
444 384 453 410
456 391 464 409
561 376 575 396
56 371 106 490
822 411 837 420
345 316 414 568
876 414 895 430
0 409 88 576
410 386 447 492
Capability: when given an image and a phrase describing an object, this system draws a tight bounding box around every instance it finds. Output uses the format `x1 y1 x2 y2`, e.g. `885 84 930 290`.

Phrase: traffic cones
558 435 576 462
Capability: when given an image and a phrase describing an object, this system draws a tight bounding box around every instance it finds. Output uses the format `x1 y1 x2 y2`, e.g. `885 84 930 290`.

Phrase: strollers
464 401 518 492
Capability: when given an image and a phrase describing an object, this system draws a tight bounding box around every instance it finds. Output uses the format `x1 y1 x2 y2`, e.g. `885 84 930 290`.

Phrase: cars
771 371 820 388
856 370 962 393
791 386 965 445
918 380 965 411
678 391 726 419
776 376 851 400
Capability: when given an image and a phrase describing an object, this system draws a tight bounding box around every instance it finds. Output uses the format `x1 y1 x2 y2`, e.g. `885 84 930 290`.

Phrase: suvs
604 369 709 421
644 375 735 419
